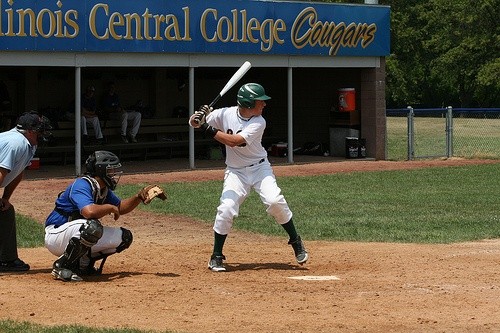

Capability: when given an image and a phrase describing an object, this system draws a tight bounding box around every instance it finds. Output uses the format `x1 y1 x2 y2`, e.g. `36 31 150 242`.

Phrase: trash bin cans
327 123 361 157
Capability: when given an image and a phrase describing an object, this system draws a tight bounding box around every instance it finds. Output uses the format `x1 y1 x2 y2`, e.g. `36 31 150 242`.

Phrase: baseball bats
193 60 253 126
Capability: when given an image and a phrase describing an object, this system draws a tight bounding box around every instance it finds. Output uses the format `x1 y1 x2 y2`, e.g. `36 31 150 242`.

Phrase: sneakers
0 257 30 271
208 252 227 272
288 235 308 263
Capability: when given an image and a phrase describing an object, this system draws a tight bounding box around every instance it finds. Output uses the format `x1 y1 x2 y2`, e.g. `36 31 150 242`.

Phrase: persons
0 78 167 282
189 83 309 271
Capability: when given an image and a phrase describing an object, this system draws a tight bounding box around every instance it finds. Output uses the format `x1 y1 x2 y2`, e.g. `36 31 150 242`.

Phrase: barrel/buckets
338 87 356 111
28 158 40 170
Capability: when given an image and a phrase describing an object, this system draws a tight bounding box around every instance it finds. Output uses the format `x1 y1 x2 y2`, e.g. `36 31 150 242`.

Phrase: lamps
177 80 186 90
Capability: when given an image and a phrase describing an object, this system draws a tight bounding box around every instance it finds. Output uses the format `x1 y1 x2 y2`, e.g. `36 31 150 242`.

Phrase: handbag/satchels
127 104 156 119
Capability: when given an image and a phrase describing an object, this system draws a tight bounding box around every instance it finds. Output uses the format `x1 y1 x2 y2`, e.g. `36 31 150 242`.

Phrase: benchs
34 119 280 166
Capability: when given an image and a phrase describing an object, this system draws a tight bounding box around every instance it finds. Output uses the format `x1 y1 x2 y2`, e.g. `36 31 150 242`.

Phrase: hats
17 114 53 132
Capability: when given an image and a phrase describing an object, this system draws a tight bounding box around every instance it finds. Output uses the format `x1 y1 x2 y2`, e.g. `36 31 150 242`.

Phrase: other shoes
80 266 98 276
120 135 129 144
51 260 83 281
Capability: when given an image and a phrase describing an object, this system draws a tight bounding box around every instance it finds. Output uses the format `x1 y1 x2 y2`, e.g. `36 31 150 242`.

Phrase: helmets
236 82 271 109
86 151 123 190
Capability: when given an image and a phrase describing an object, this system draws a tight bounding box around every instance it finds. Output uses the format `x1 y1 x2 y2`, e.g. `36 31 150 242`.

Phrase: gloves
195 105 213 125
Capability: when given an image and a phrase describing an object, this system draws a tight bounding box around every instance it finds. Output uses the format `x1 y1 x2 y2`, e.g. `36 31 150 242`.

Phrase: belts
250 158 264 166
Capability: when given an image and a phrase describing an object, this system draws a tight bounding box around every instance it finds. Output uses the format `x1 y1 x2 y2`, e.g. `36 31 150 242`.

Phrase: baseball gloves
138 184 168 205
